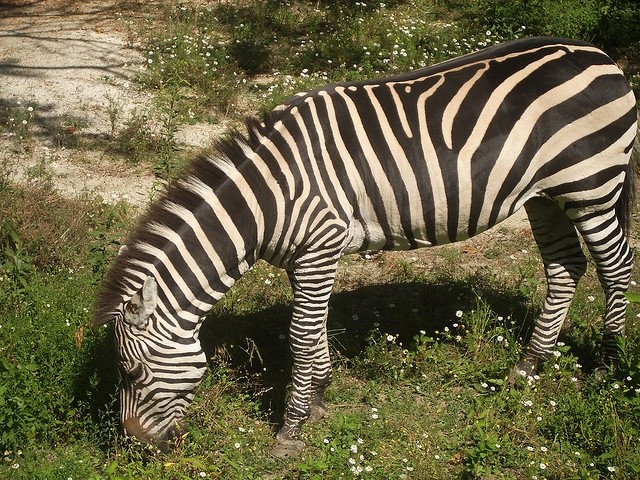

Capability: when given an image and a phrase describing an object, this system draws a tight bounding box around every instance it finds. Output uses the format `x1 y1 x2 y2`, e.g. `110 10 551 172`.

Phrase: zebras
89 37 640 461
